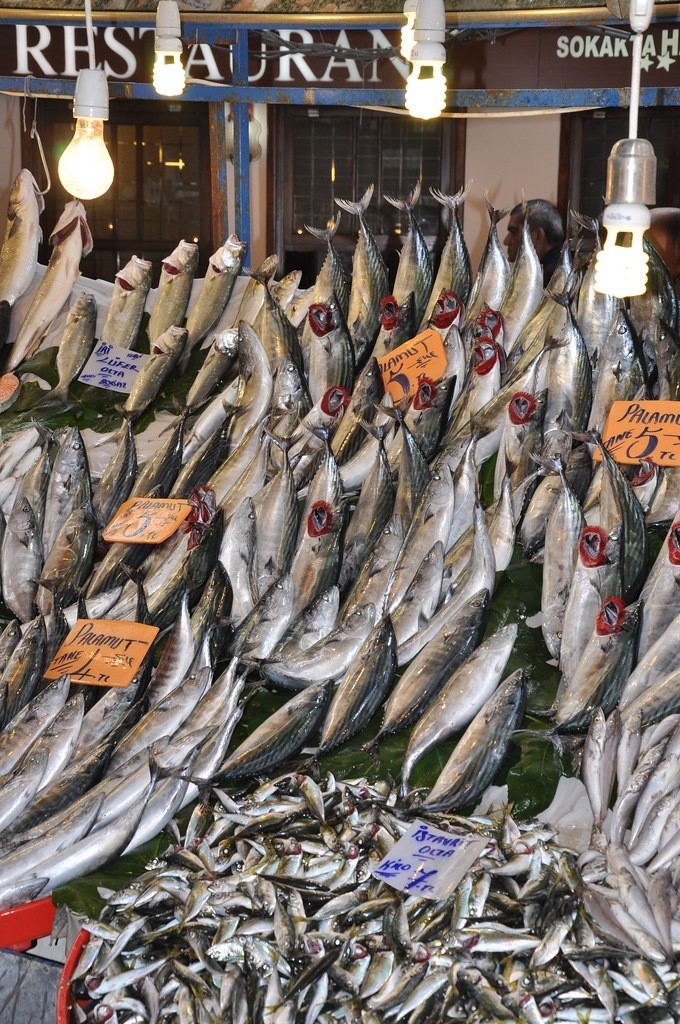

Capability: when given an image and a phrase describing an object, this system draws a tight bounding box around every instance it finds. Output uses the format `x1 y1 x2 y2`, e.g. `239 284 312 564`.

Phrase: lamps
400 0 448 121
152 0 186 97
56 0 116 201
595 0 658 298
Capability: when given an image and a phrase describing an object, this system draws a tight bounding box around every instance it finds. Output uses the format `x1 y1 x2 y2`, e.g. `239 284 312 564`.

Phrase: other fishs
0 167 680 1024
